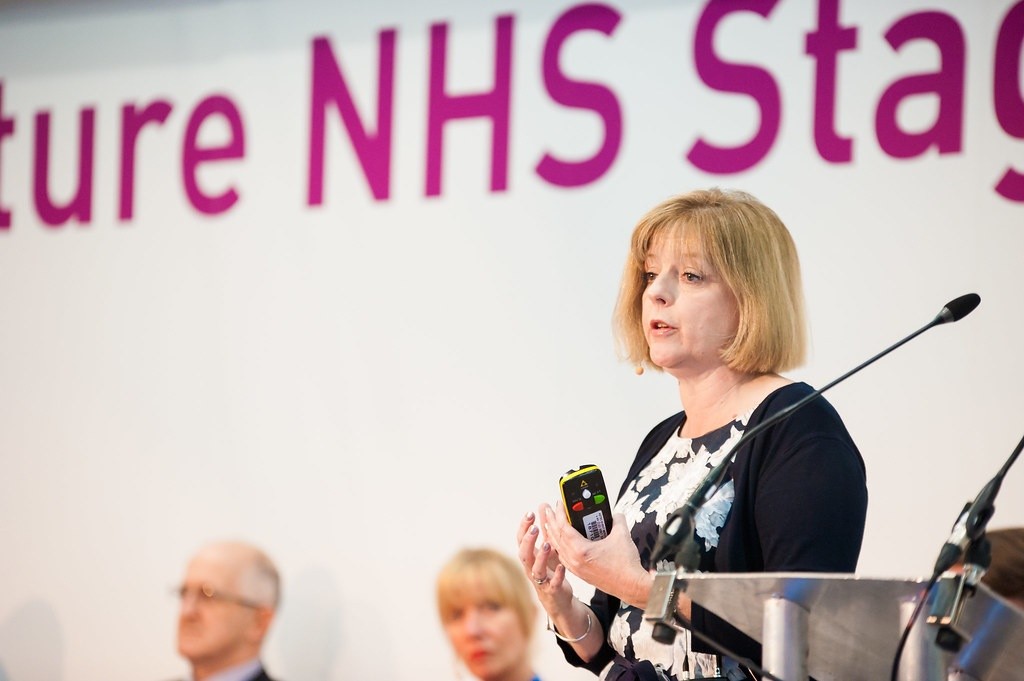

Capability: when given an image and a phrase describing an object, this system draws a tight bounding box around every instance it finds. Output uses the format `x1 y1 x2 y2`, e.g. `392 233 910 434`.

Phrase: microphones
651 293 982 564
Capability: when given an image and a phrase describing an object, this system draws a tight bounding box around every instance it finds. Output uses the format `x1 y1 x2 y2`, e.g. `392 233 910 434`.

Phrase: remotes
560 462 614 541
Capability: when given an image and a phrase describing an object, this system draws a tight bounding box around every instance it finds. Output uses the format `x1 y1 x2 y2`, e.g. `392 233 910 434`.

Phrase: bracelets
546 611 592 643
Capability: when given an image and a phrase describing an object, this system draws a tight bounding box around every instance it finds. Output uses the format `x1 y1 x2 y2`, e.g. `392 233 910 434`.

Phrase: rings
532 574 549 584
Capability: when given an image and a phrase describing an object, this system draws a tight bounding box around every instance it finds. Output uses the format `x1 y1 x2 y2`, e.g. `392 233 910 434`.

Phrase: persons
431 550 549 681
520 185 867 680
172 538 281 681
